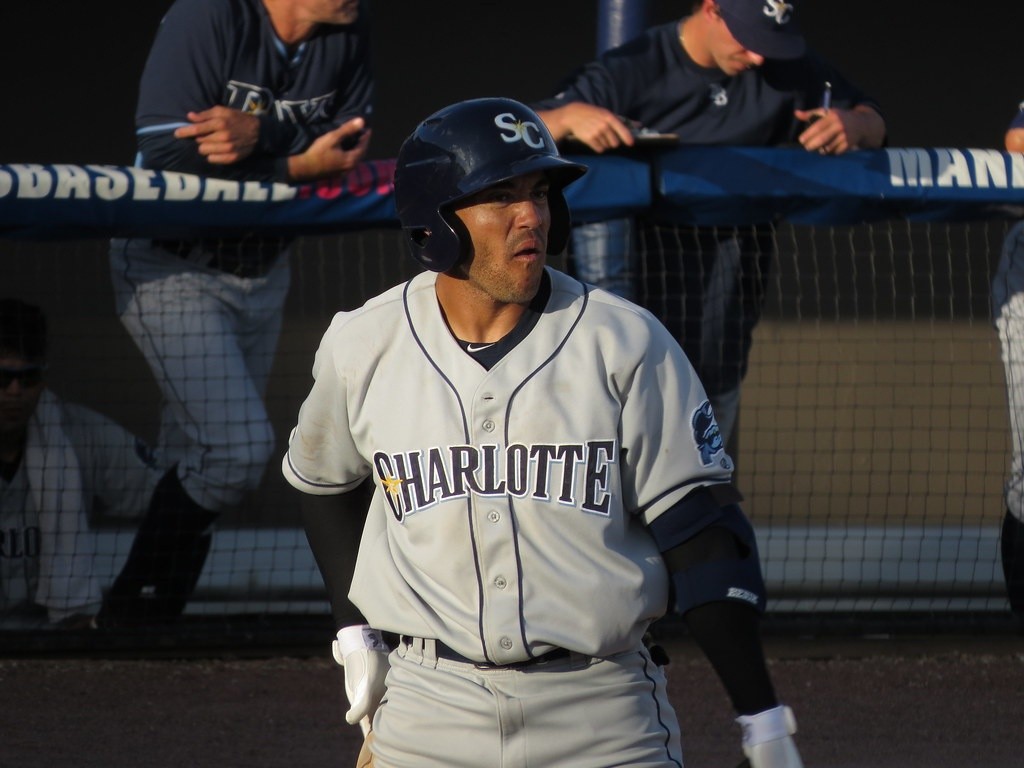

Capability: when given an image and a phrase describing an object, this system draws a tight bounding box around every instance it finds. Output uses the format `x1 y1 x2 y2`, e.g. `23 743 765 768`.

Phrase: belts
151 236 285 276
402 633 574 670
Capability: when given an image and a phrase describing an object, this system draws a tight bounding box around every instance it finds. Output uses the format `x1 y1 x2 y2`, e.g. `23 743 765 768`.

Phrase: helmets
395 99 589 274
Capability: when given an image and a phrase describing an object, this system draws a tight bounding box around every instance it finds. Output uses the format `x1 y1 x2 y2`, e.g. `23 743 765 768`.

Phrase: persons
0 297 164 629
90 0 379 629
532 0 888 504
991 100 1024 647
281 98 805 768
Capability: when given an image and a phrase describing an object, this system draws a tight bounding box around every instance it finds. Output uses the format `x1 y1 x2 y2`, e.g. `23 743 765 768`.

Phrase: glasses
0 367 45 389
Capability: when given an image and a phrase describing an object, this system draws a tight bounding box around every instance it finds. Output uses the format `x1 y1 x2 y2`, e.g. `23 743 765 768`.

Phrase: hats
715 0 807 60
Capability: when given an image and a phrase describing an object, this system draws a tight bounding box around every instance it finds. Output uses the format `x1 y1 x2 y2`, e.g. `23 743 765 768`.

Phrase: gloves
734 704 804 768
332 625 392 740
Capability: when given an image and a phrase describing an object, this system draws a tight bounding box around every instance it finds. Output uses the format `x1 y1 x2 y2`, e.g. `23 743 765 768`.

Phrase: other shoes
61 616 135 661
